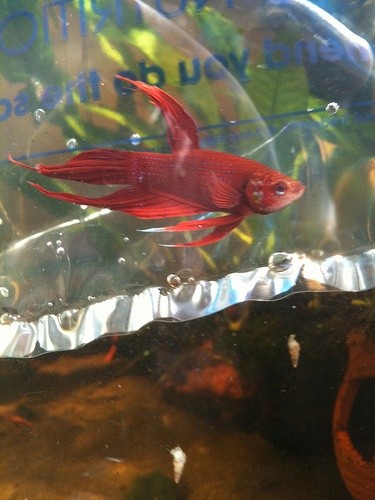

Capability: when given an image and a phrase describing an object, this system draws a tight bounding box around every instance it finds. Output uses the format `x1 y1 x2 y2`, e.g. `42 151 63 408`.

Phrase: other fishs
7 74 304 249
